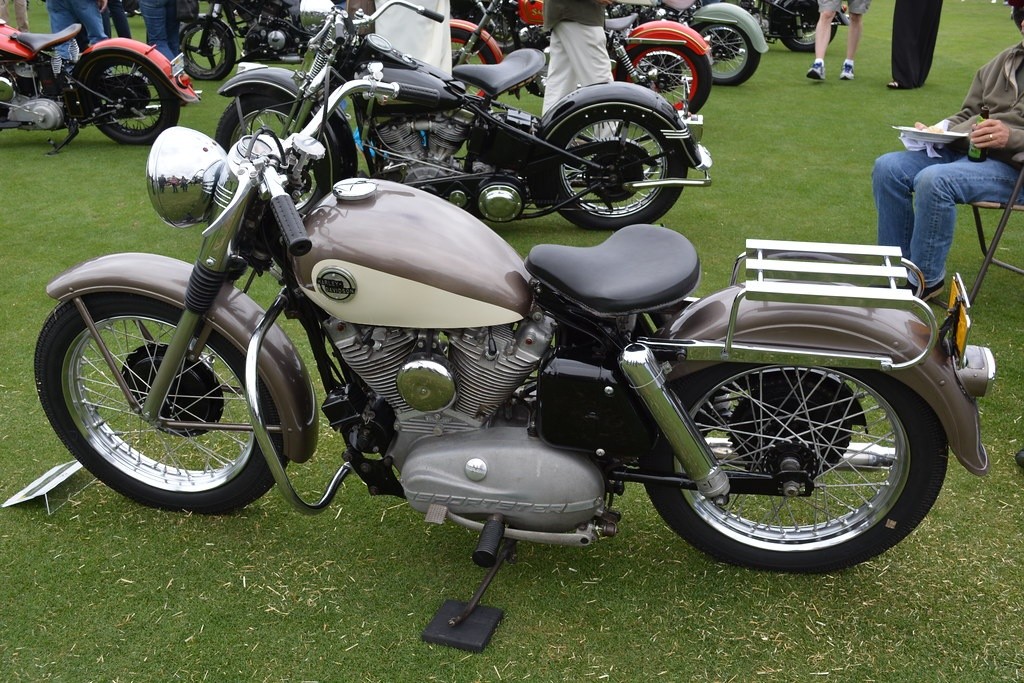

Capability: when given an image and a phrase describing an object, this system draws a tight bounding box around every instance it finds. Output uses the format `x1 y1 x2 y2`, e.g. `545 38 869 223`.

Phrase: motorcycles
0 19 203 161
177 0 342 83
448 0 851 121
214 0 712 233
32 68 999 652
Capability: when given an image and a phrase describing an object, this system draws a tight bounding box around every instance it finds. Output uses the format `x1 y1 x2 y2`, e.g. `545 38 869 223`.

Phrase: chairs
926 152 1024 310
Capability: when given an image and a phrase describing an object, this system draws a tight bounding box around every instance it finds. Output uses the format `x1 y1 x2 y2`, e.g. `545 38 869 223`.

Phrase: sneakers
840 59 854 80
806 60 824 81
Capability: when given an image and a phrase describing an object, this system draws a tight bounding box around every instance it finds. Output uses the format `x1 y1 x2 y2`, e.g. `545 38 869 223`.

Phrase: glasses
1011 11 1024 20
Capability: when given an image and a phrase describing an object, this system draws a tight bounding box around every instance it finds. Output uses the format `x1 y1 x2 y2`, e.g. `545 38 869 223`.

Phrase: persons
542 0 621 141
871 0 1024 302
1 0 193 106
806 0 871 80
886 0 943 89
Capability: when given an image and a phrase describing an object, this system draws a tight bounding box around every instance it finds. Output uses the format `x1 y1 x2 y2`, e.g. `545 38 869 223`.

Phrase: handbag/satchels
176 0 199 23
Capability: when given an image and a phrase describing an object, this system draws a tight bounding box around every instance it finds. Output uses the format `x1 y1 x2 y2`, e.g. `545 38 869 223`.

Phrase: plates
892 125 970 144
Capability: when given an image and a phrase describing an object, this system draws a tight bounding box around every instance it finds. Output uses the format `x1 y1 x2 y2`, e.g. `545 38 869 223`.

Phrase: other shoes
887 81 907 88
868 278 946 302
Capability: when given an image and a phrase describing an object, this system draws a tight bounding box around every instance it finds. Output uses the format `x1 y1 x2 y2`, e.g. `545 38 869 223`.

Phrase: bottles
968 106 989 162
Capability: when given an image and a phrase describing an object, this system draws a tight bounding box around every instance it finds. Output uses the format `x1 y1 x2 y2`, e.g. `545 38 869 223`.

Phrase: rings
990 134 992 140
